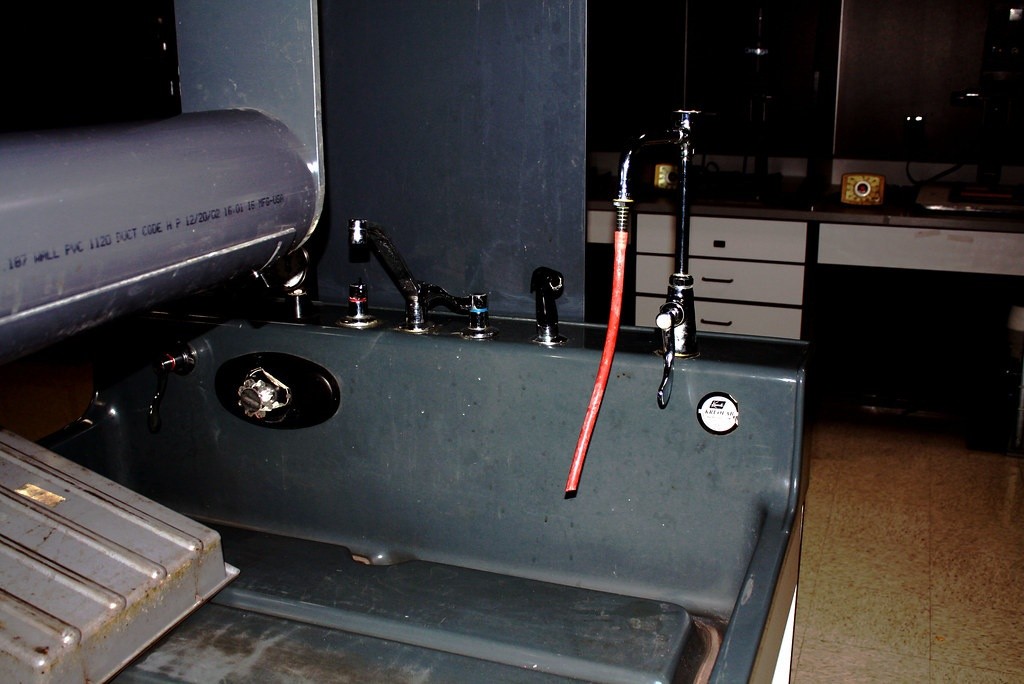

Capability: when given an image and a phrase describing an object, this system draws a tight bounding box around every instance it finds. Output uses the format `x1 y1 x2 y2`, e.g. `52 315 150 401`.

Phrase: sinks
38 301 812 613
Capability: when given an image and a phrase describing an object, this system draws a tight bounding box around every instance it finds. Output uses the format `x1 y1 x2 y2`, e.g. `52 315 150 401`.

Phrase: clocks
840 172 885 206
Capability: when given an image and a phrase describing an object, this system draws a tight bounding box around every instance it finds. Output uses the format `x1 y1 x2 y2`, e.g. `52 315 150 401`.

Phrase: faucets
344 217 430 333
611 103 704 409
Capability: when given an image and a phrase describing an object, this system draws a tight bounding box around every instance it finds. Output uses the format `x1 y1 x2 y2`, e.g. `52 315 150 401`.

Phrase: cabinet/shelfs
586 180 1024 453
